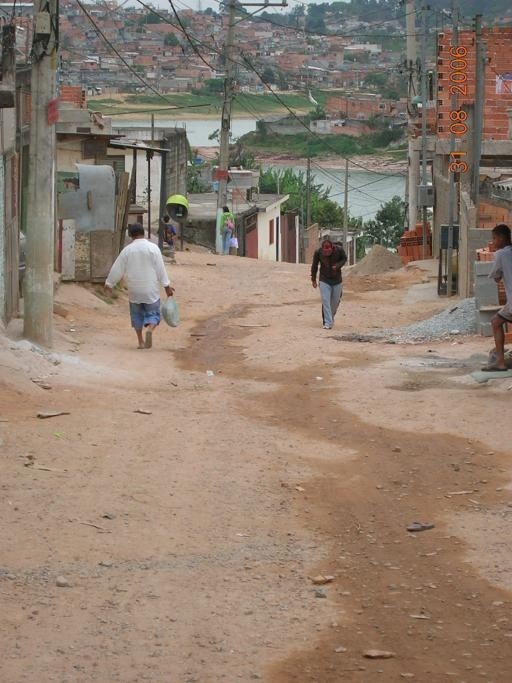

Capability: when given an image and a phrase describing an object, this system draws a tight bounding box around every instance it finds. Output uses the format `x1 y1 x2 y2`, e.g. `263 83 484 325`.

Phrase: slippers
145 330 152 348
482 362 507 370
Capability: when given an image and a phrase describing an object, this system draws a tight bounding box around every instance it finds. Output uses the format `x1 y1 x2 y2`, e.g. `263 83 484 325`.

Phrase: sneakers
323 324 331 328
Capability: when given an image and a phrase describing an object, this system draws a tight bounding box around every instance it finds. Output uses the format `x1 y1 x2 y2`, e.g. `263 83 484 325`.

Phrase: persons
220 206 235 255
310 239 347 329
105 222 176 349
480 223 512 372
163 214 176 250
